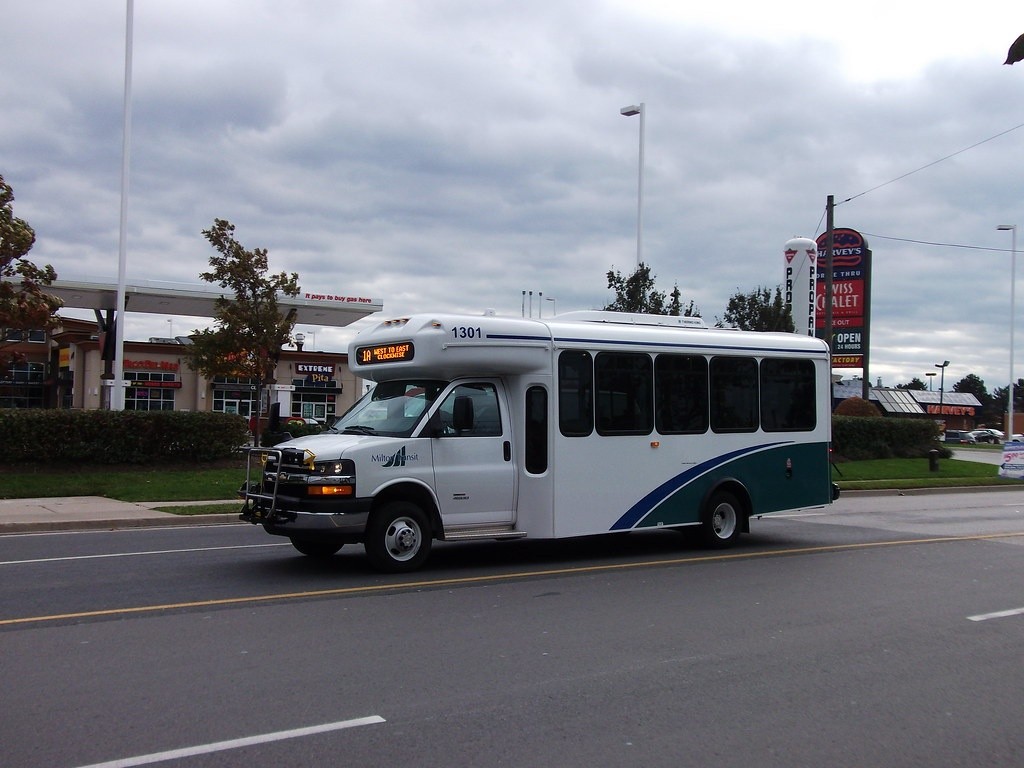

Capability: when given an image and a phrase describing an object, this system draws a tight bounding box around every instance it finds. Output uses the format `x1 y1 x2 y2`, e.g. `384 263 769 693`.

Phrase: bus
239 307 841 573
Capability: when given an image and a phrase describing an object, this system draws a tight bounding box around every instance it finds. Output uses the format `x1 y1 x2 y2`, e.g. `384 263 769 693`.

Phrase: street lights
995 223 1016 443
935 361 950 413
307 331 316 351
167 320 173 338
925 373 937 391
546 298 556 316
621 102 647 271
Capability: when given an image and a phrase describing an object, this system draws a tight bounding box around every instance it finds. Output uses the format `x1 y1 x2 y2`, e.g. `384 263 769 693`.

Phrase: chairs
464 394 498 437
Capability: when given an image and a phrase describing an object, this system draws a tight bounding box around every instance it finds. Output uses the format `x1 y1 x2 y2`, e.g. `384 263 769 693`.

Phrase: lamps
293 333 305 352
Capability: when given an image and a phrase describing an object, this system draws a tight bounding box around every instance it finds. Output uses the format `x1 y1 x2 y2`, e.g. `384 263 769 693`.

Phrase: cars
1012 434 1024 443
304 418 326 429
946 430 976 445
933 431 946 442
971 430 1001 444
974 429 1005 444
